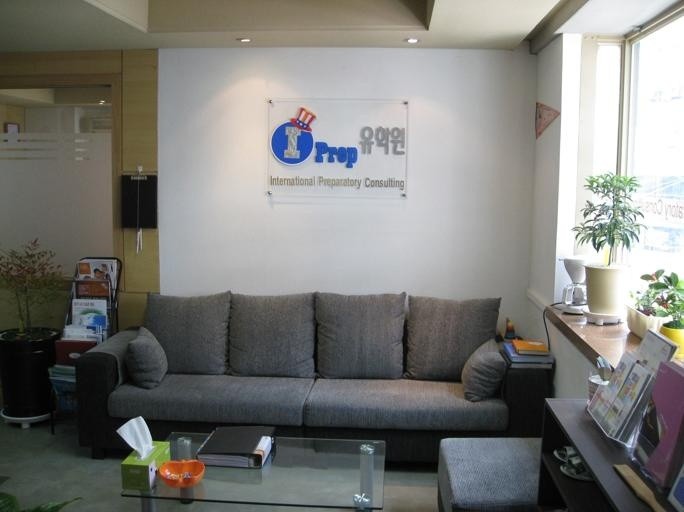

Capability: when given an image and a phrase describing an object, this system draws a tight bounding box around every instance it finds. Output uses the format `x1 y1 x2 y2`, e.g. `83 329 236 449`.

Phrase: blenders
542 257 589 352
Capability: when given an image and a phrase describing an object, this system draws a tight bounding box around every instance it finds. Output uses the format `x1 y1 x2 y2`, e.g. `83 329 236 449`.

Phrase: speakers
121 174 157 229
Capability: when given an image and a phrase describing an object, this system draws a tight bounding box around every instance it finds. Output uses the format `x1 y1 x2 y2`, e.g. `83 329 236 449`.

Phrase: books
587 328 679 444
49 259 118 409
500 340 554 370
628 361 684 492
668 466 684 512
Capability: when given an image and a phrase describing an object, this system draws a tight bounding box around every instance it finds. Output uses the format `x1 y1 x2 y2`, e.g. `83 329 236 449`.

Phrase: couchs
77 288 550 472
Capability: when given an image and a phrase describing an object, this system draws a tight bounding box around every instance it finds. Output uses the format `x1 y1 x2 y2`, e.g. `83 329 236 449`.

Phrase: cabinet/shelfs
539 394 682 512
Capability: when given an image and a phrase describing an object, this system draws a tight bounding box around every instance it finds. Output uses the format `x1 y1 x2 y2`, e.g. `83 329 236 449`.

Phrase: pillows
315 290 406 379
129 327 168 390
144 290 232 375
406 292 501 380
229 293 316 380
461 338 505 402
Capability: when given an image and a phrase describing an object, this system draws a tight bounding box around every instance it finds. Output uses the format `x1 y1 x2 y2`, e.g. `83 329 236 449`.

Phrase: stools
437 435 547 512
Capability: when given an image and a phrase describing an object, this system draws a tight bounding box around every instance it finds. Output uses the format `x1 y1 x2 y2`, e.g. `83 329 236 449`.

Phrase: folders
197 425 275 470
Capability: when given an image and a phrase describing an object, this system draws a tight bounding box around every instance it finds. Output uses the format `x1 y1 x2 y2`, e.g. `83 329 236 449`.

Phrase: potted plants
654 275 684 362
572 167 647 327
628 268 673 340
1 239 73 429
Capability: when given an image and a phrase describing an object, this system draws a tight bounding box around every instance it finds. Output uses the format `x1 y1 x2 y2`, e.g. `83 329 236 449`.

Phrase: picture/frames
5 123 20 142
